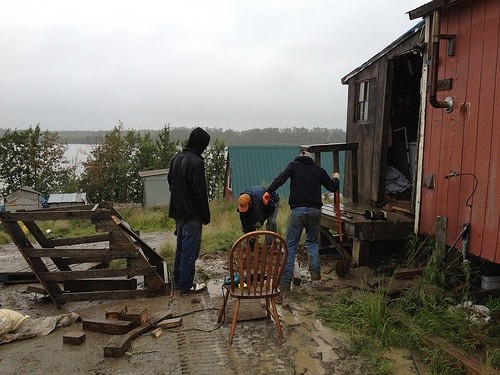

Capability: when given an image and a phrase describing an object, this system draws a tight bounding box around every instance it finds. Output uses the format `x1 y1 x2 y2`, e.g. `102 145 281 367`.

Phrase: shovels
335 170 352 278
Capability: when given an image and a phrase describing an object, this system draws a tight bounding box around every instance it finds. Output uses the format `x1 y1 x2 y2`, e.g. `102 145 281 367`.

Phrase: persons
168 128 210 293
263 152 340 293
237 185 280 254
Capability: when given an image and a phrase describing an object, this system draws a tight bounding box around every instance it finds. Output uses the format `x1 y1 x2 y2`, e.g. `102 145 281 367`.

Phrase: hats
238 194 251 212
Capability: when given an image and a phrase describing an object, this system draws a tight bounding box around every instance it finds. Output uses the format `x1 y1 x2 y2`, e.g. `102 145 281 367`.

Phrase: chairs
217 231 289 345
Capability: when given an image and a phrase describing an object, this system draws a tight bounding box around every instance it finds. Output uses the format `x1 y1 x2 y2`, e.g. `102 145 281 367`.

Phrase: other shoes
180 283 206 297
